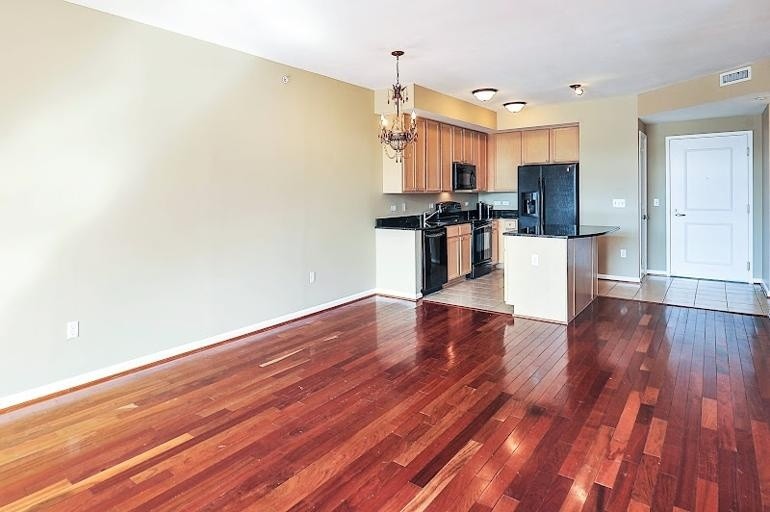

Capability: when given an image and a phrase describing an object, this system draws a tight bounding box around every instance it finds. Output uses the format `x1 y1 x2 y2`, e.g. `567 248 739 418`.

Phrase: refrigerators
516 163 578 235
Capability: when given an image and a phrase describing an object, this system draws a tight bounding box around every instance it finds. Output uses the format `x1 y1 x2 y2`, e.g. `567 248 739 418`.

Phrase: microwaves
452 162 478 190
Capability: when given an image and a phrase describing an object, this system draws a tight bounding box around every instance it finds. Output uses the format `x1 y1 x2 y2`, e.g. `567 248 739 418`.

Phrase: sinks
426 222 448 228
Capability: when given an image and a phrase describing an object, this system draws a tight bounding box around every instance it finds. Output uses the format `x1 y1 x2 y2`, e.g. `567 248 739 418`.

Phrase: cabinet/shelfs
444 223 472 281
494 126 578 193
382 113 488 194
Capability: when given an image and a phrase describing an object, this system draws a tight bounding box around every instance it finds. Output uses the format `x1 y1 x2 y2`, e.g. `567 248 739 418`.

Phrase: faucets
423 210 439 222
423 222 432 228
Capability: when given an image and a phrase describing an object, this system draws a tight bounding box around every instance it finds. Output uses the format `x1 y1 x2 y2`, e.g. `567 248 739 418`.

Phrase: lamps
472 89 527 113
378 50 418 164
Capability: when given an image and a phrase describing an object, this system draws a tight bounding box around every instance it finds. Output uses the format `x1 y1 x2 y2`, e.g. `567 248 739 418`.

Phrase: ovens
471 221 493 266
